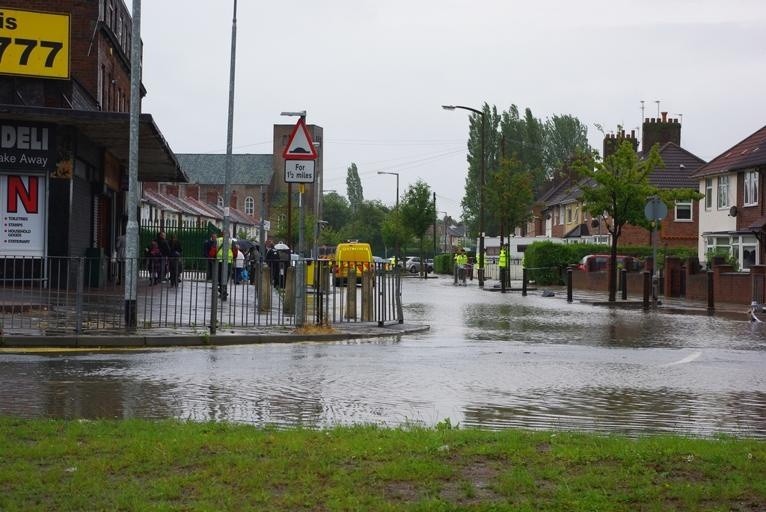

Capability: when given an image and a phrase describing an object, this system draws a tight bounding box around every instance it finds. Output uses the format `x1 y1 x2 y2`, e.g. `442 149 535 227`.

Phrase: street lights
278 108 320 330
441 104 486 287
376 170 399 273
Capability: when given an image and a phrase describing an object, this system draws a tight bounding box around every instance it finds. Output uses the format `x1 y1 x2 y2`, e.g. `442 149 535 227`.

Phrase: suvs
575 253 656 273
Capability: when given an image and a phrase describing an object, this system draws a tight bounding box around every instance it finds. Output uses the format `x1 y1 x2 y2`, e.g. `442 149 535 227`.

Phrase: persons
115 225 281 300
454 243 509 288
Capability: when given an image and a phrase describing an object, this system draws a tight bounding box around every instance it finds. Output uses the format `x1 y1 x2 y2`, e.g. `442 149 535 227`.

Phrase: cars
274 243 434 275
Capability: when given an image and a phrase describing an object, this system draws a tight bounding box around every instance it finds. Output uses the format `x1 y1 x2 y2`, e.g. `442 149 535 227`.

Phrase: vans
330 239 377 289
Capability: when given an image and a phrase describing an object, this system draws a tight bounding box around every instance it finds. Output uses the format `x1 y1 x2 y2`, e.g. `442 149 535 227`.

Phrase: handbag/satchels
241 269 250 282
209 243 223 257
